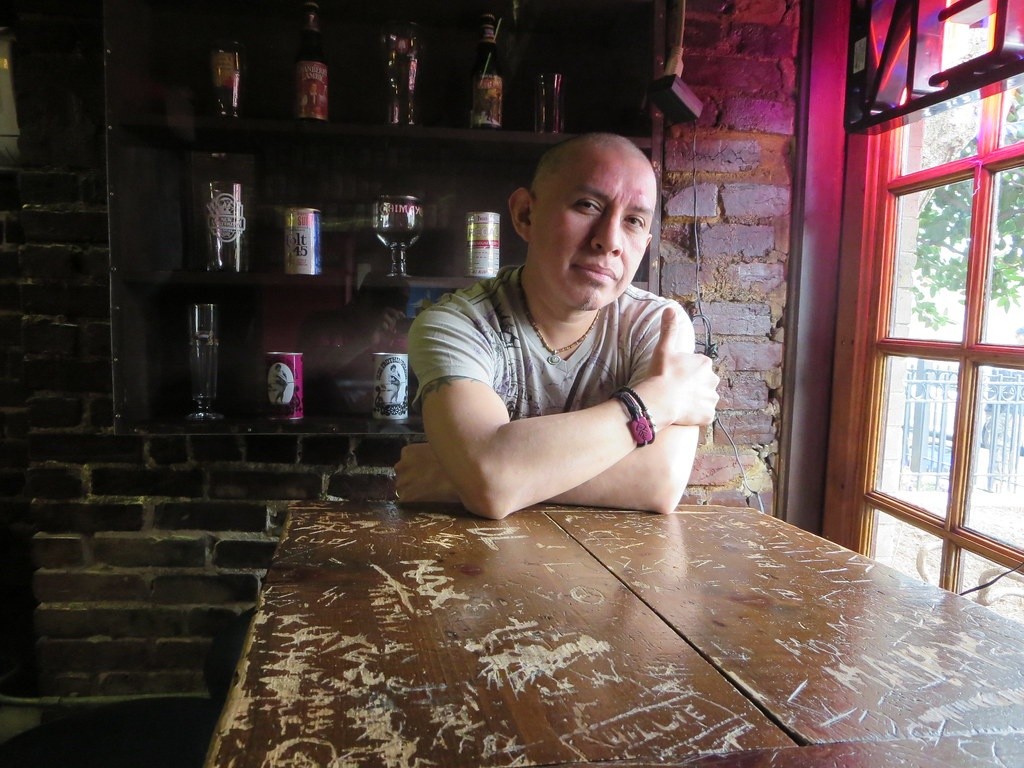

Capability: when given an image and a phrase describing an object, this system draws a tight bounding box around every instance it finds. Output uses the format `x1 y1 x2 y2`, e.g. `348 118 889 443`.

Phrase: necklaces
515 276 601 365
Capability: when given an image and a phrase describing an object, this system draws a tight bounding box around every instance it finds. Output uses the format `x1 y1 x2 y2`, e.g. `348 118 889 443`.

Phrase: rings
395 489 401 499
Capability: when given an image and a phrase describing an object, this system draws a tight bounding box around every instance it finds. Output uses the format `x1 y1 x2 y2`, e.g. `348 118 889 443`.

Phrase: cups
373 352 410 421
377 18 424 126
210 39 250 119
264 352 304 421
202 180 251 274
532 72 566 134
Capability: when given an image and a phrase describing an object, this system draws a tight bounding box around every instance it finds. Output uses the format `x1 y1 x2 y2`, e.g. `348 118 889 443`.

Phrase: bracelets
609 387 655 447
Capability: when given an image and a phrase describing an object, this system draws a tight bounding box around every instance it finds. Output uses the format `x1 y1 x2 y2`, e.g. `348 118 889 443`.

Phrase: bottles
292 3 330 122
468 13 504 131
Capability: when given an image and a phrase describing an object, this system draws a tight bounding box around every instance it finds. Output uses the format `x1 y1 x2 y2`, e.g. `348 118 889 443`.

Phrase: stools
0 697 225 768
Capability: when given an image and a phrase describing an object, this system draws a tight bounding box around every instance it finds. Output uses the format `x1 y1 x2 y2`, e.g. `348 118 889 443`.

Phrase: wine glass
187 303 225 420
372 193 424 277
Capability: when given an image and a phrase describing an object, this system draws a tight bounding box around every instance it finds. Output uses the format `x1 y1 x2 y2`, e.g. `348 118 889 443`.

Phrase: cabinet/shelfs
101 0 668 440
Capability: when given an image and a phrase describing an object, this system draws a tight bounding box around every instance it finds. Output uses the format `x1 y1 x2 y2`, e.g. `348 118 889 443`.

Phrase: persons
391 131 720 519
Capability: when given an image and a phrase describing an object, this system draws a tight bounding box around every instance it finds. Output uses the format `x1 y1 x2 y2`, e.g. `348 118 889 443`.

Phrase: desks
201 499 1024 768
